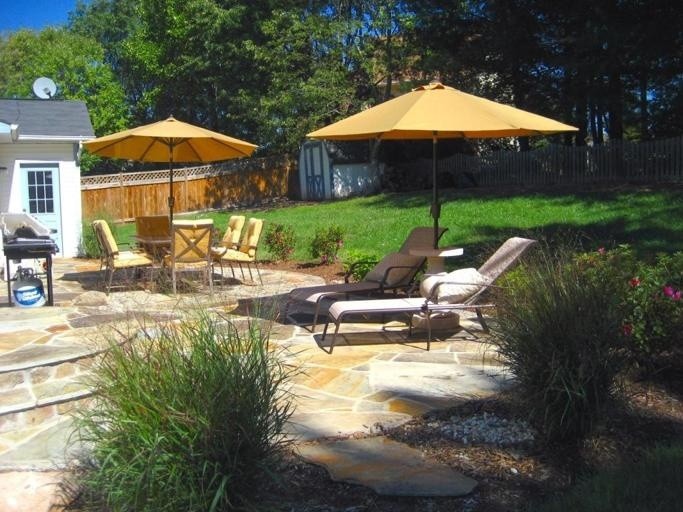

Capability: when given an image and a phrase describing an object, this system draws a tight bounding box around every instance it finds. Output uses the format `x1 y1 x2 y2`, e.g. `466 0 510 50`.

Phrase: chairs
92 214 264 296
284 226 449 332
321 236 538 354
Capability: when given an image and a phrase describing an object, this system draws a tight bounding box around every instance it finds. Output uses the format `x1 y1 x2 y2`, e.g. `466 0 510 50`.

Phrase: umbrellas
82 113 258 228
306 81 580 247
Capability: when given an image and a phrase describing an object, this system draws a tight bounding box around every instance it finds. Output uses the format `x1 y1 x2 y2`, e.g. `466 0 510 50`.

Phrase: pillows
432 268 488 306
365 254 421 287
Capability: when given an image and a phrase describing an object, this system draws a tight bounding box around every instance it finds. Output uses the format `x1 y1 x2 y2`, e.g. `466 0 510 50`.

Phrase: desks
410 247 463 330
